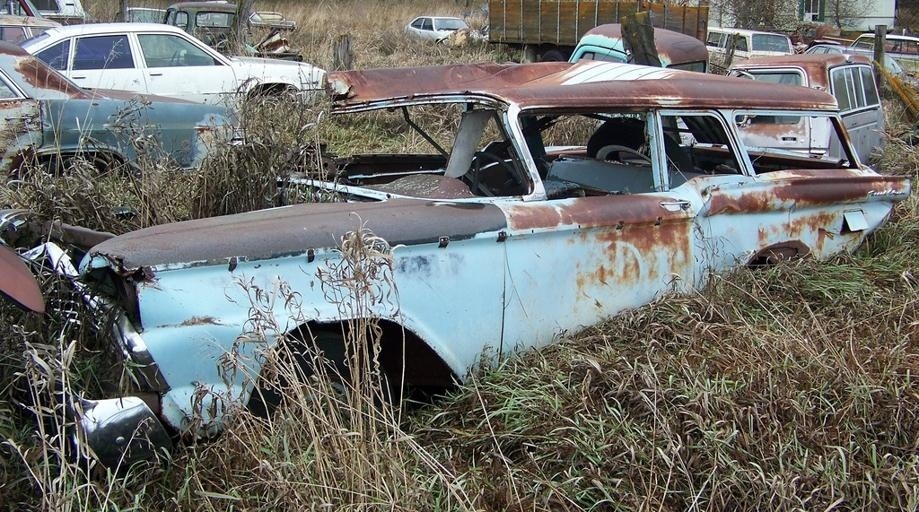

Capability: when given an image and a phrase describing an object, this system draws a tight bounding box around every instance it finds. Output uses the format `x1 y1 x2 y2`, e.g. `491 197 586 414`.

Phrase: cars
109 1 300 61
703 25 795 72
405 15 472 46
800 32 918 82
0 39 236 184
695 55 889 172
0 0 87 56
17 22 329 116
567 24 709 74
